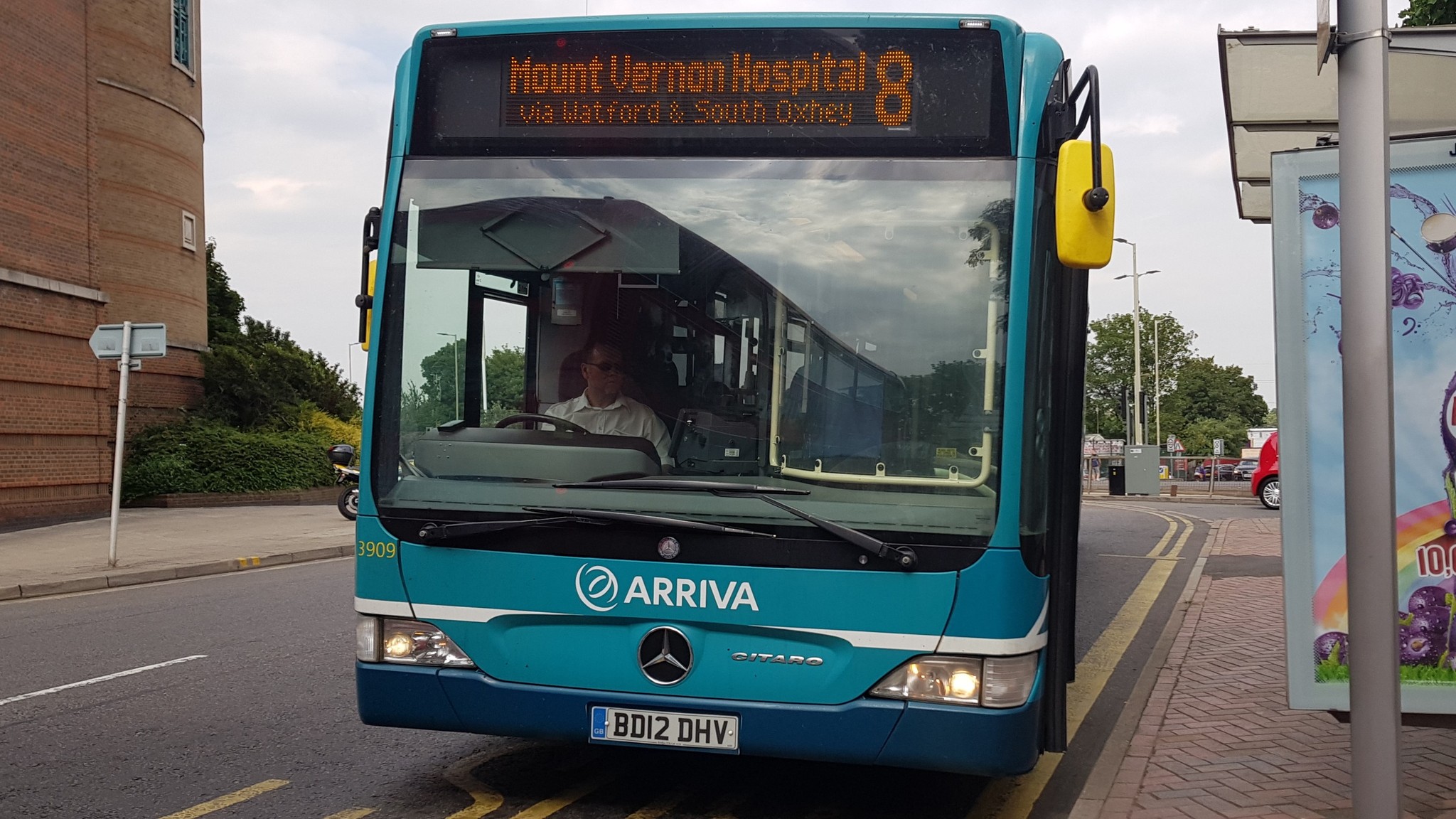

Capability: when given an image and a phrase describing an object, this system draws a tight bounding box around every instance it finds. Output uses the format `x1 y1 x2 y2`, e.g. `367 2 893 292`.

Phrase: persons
779 312 883 474
1091 451 1101 481
541 340 675 477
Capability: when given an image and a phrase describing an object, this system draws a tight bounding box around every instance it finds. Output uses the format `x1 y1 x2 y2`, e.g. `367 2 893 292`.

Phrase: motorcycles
327 444 417 520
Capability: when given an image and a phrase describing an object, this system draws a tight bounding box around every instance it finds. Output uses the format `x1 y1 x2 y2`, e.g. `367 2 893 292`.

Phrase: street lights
349 342 359 384
1114 270 1162 446
1113 238 1140 445
1155 317 1177 448
436 332 459 420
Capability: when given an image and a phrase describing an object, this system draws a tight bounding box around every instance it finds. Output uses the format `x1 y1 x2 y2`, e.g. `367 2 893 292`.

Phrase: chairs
557 346 864 463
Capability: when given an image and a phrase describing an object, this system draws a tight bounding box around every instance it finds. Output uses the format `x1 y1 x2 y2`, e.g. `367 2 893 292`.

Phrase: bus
355 7 1117 779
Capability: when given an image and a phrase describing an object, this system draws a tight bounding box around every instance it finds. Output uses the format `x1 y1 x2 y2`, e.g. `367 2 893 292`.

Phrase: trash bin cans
1108 465 1125 496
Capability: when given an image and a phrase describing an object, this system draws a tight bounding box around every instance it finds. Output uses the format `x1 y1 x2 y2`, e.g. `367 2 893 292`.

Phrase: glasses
586 360 630 373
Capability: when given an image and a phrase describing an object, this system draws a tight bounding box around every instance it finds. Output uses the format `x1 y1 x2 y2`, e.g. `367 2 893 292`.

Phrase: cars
1203 464 1236 481
1251 433 1281 510
1234 457 1259 482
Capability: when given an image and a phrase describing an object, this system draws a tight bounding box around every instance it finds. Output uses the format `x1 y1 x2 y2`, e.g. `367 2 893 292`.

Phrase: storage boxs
327 442 355 468
